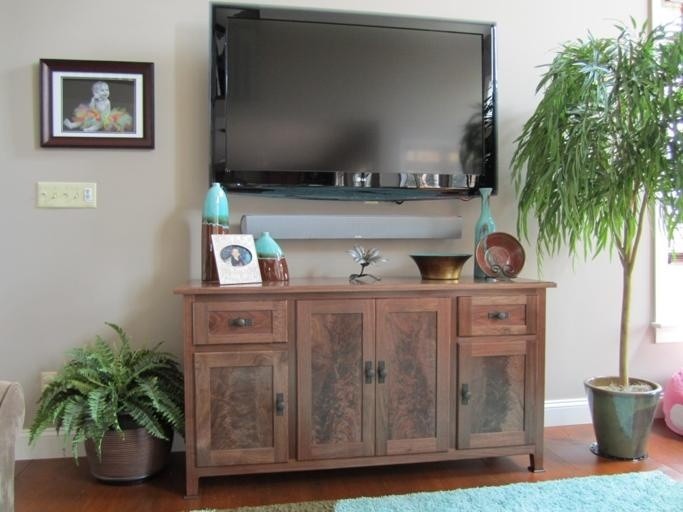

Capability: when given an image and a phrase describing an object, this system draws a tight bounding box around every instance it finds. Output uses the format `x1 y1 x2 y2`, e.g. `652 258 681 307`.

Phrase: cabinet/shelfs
455 282 555 472
294 286 454 472
184 288 291 496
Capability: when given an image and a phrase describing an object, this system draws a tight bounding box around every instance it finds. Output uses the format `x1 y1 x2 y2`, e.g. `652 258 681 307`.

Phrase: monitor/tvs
208 2 498 198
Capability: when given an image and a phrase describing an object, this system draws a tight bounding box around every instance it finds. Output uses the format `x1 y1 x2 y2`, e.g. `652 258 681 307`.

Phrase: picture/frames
210 233 263 286
38 58 155 150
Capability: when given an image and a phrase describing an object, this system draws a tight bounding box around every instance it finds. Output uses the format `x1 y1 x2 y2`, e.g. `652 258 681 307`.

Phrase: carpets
188 470 682 511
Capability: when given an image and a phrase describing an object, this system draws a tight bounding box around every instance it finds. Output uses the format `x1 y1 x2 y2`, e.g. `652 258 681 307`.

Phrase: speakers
240 213 465 241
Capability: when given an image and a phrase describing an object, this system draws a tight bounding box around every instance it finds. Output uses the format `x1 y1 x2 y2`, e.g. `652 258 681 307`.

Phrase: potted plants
27 318 185 483
507 14 682 462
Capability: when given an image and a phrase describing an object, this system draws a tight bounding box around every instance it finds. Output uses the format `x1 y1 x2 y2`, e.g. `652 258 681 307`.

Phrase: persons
62 80 113 132
225 247 247 267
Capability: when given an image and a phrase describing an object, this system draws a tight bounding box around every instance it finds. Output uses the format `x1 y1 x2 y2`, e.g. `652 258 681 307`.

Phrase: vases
472 186 497 280
199 180 228 282
254 231 289 282
